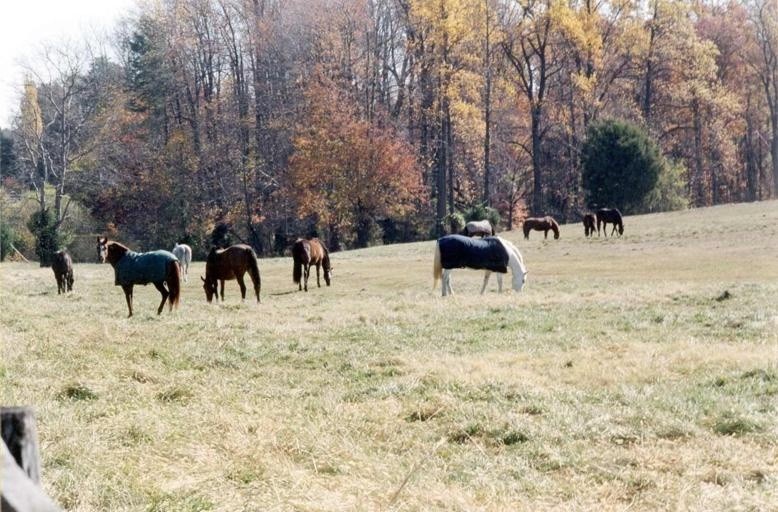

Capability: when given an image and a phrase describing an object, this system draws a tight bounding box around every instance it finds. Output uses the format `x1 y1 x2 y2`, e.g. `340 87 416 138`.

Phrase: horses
522 213 561 240
51 248 74 295
169 239 192 283
430 233 530 296
290 236 333 291
200 243 261 302
461 219 495 238
581 206 625 238
95 236 180 318
